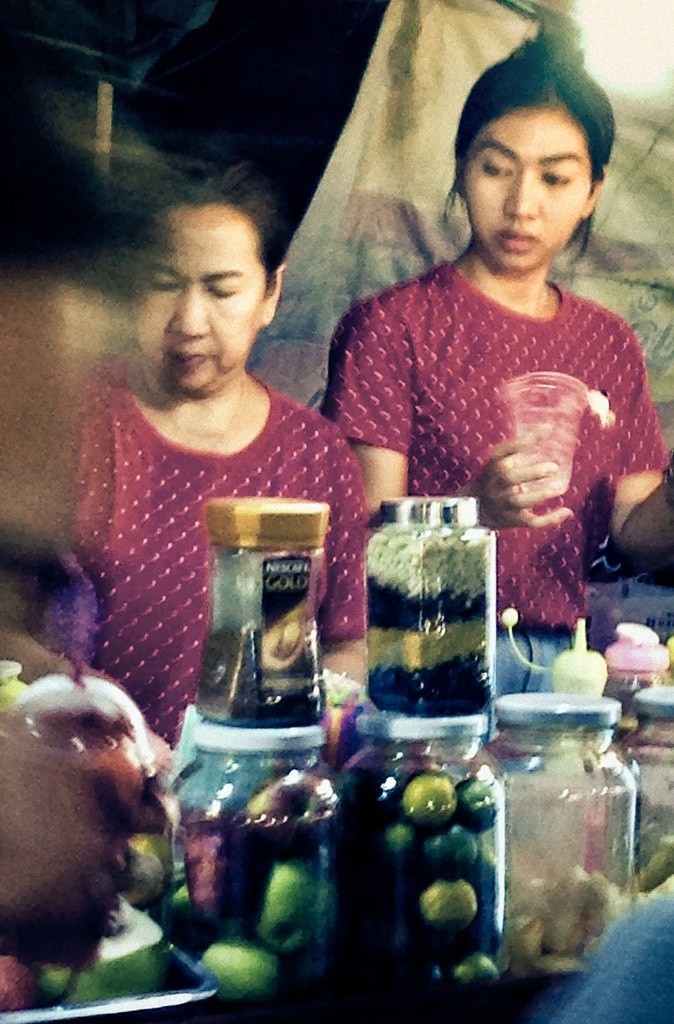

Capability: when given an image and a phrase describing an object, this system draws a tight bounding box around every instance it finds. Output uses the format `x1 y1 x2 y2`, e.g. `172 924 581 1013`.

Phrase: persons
0 47 174 944
322 34 674 697
0 159 369 749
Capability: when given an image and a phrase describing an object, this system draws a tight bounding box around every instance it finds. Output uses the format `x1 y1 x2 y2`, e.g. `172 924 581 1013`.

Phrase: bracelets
664 449 674 507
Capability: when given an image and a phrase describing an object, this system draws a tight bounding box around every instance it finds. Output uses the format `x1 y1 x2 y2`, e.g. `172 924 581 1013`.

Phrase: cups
504 371 591 497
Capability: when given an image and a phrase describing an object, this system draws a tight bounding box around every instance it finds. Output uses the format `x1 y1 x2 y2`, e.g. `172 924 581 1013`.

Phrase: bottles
163 497 673 1002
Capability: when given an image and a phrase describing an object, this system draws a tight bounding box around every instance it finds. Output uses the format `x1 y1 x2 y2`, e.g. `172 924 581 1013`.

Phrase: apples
163 773 339 1003
